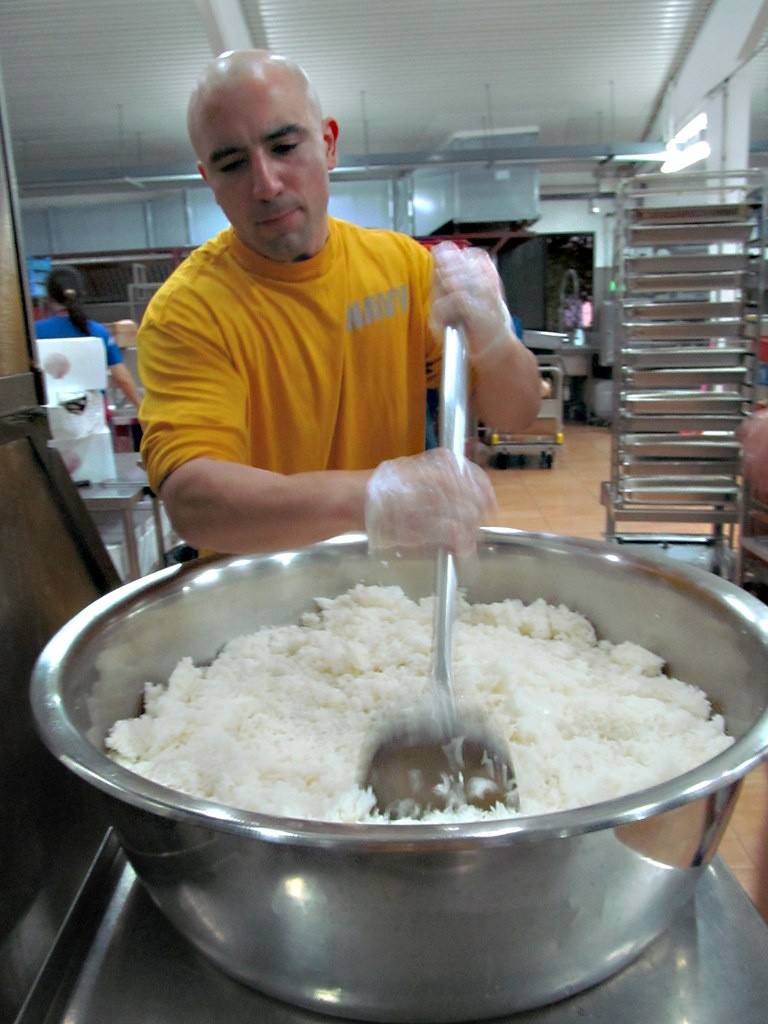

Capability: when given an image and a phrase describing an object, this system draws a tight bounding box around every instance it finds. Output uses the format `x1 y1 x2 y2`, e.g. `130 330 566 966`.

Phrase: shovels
364 312 525 819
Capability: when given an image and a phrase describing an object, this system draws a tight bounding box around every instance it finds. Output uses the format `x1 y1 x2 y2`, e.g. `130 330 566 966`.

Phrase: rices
101 585 736 825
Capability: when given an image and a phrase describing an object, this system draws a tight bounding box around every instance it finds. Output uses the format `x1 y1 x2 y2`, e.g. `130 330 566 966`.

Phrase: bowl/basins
29 524 767 1022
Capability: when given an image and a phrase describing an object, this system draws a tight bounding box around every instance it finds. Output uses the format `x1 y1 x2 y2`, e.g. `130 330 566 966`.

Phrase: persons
138 48 537 560
34 267 142 449
